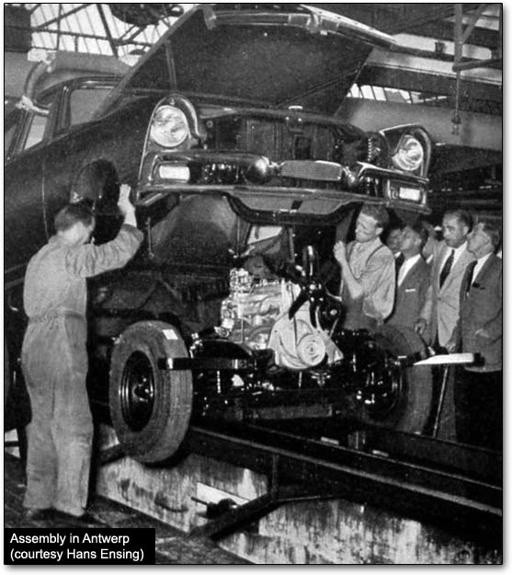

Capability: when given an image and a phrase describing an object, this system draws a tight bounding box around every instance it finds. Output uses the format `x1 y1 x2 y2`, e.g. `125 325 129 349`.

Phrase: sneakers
25 509 109 529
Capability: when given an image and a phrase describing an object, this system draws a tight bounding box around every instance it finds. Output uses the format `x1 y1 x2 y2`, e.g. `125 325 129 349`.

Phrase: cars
3 3 435 467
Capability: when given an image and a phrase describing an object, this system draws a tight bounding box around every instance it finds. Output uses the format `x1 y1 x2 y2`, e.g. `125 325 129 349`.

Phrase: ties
465 259 480 292
439 249 455 288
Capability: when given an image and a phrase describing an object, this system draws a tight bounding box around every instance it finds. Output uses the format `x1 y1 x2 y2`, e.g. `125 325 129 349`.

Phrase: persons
384 222 404 276
20 181 147 529
419 218 438 260
412 205 478 443
443 217 503 455
331 202 397 335
380 218 435 436
